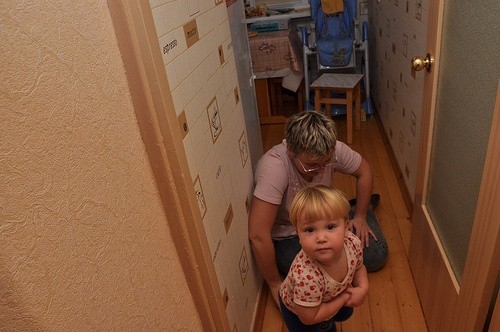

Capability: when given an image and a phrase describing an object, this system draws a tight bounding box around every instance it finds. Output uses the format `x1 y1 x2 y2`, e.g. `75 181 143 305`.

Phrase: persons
248 109 390 316
279 182 371 332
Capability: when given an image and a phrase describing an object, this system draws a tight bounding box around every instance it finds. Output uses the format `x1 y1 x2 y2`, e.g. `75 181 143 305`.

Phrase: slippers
349 193 380 210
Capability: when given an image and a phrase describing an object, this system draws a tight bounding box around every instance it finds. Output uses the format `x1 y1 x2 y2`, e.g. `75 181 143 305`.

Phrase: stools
310 73 364 145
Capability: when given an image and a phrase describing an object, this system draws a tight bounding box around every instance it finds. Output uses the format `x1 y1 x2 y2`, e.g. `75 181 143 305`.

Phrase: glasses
298 152 339 172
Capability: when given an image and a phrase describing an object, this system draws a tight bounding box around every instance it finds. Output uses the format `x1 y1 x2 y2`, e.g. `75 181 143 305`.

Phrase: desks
249 23 304 124
